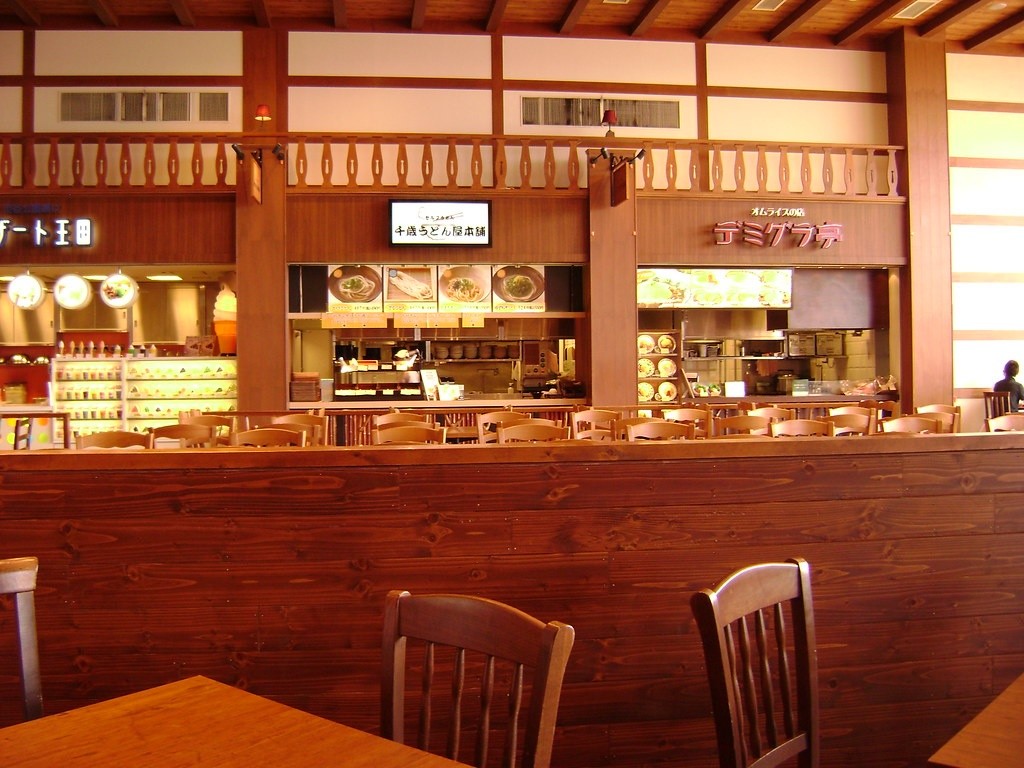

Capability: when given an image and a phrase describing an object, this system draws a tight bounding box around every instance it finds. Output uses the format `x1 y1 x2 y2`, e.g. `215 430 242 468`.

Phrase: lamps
254 105 272 127
272 143 285 165
601 110 618 138
628 149 646 168
590 147 610 169
231 144 245 165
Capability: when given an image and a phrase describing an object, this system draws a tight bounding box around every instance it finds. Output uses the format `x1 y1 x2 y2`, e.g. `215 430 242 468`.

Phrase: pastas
389 270 432 300
338 274 376 302
500 275 536 301
445 277 483 301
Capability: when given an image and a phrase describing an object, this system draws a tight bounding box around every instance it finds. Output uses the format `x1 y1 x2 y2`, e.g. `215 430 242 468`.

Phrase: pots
775 374 799 391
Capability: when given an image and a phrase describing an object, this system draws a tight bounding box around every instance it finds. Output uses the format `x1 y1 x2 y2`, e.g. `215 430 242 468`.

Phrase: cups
698 343 707 357
57 367 124 439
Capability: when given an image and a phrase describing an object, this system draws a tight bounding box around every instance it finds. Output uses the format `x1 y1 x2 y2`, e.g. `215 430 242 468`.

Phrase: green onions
504 276 534 297
459 278 475 298
342 278 364 291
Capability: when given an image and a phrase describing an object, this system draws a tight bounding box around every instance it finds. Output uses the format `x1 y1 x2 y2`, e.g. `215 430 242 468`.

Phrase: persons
993 360 1024 417
394 344 420 370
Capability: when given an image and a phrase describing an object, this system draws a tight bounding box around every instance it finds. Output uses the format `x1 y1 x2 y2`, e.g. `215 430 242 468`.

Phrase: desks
928 672 1024 768
441 426 489 443
0 675 469 767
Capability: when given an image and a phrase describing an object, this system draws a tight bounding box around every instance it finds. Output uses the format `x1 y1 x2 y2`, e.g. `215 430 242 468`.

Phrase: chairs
75 400 963 450
379 590 576 768
984 391 1010 419
689 558 820 768
985 415 1024 431
14 417 33 450
0 556 45 720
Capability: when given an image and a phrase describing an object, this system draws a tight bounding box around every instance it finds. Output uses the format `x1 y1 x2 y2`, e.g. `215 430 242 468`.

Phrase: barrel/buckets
3 383 27 404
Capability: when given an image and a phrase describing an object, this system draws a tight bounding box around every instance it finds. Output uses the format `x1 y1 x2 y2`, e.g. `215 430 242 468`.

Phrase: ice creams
213 282 239 354
58 340 157 357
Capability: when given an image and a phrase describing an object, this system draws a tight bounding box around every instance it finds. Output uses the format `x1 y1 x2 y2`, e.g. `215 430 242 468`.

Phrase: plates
692 384 722 395
637 334 678 401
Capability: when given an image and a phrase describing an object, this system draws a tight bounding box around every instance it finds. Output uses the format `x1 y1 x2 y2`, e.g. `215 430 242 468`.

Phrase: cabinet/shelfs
50 356 237 450
637 328 682 406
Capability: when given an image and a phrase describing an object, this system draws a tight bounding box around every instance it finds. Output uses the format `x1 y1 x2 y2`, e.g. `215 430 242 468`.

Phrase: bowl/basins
329 265 383 303
438 264 491 303
530 392 543 398
434 344 520 359
492 265 545 302
33 397 49 405
